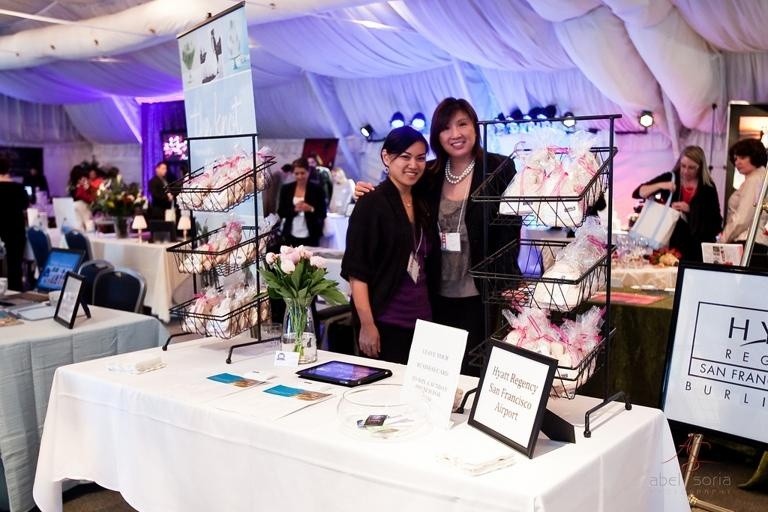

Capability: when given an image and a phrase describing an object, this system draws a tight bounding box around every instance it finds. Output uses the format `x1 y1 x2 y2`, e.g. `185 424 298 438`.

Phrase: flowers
70 172 104 203
259 243 343 304
89 173 151 215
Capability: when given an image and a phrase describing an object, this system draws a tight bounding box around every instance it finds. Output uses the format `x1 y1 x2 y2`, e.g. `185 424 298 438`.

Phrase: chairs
0 225 147 315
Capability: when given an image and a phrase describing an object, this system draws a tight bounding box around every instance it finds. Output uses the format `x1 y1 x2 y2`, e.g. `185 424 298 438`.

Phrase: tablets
296 361 392 387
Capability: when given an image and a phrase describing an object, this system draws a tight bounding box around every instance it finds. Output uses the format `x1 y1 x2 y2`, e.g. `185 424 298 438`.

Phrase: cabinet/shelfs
454 113 633 438
160 133 283 362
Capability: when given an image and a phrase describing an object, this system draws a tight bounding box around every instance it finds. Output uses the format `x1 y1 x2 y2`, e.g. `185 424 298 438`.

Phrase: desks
515 277 675 408
0 286 170 512
29 213 203 322
33 331 697 512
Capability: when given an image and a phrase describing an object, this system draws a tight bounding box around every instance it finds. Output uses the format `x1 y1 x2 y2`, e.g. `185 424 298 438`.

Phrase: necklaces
682 177 696 194
445 157 476 184
406 202 412 208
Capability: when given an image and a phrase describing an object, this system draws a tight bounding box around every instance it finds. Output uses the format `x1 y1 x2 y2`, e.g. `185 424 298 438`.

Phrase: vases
110 216 131 238
279 302 320 364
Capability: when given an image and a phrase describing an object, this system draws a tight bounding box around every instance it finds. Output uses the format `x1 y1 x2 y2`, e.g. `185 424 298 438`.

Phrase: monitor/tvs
160 131 189 163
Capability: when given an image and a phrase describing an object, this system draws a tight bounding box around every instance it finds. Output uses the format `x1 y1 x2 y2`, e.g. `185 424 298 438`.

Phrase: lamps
484 106 657 135
126 208 192 245
357 109 430 144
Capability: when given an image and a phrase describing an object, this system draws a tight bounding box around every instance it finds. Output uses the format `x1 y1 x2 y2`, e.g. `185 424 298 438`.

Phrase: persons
279 159 326 247
24 166 50 201
631 146 722 253
68 166 139 216
340 126 439 365
353 96 522 376
281 152 354 214
148 162 172 217
0 148 28 294
716 139 768 272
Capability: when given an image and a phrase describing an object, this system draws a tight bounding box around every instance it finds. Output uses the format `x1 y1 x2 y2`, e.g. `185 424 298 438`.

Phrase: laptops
0 248 86 306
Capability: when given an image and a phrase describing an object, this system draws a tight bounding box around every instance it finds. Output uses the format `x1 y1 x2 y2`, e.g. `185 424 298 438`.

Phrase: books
0 294 57 321
198 356 332 423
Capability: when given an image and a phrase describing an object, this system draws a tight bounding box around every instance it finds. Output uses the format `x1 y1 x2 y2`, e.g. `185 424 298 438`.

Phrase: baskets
162 155 277 340
466 145 617 399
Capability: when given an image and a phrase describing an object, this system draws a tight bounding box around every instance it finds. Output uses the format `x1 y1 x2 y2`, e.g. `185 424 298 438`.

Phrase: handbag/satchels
628 199 682 251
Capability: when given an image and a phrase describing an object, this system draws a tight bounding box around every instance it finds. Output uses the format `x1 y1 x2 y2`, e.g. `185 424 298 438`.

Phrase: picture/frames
53 270 91 328
467 333 575 460
659 257 767 453
